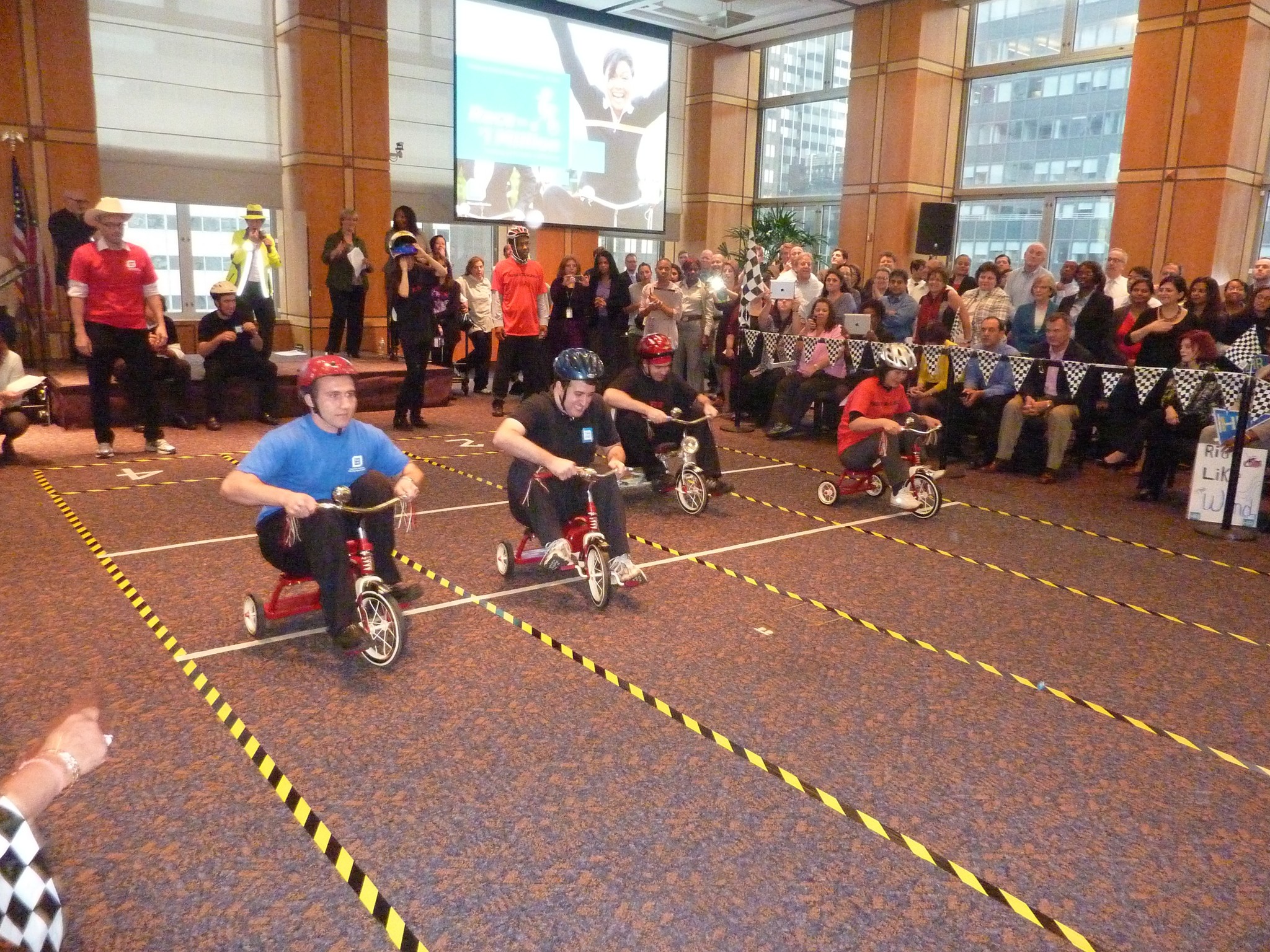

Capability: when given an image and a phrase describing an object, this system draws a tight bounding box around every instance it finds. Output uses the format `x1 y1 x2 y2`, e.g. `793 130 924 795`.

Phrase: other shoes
453 364 522 417
134 408 280 432
949 433 1167 502
706 382 836 437
2 442 18 461
347 352 360 359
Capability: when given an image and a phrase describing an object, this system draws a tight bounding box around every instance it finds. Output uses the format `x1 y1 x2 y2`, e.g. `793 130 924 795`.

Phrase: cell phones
650 287 651 295
810 315 817 327
575 276 581 281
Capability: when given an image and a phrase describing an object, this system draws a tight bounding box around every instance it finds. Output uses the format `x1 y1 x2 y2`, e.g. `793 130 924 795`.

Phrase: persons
383 205 467 431
835 344 945 509
48 188 96 366
222 354 424 650
116 293 198 431
0 706 107 952
64 197 175 458
492 347 647 587
0 316 29 462
456 226 1270 500
321 210 373 359
603 333 735 496
543 15 668 231
226 204 283 362
197 281 278 429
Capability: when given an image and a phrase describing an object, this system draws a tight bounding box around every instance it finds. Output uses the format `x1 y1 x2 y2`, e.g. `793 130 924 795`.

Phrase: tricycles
633 407 712 515
241 485 414 670
816 424 943 519
494 464 635 608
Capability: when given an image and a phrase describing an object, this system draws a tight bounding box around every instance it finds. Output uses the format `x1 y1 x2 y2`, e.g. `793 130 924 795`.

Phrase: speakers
915 202 956 256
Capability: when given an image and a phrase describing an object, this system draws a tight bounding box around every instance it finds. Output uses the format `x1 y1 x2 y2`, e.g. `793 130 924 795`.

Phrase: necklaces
1159 303 1182 322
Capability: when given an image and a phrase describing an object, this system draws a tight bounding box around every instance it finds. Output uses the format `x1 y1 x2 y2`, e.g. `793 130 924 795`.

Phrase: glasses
1106 257 1125 264
68 197 88 208
97 221 125 230
876 276 889 281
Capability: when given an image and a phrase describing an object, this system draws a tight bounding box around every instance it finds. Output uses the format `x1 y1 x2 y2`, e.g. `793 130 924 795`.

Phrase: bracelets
814 364 820 370
43 749 81 798
1047 400 1051 409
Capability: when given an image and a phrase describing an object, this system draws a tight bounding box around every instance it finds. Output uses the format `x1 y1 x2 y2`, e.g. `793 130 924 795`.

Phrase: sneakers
705 478 734 495
94 439 175 459
334 582 422 645
889 464 946 510
651 474 676 491
393 414 427 431
540 539 643 586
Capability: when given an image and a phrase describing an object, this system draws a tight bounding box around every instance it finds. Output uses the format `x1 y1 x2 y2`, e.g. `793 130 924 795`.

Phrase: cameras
235 326 243 333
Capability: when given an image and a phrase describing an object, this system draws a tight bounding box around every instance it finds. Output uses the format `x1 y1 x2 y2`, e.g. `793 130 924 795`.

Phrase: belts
713 316 722 320
680 315 702 321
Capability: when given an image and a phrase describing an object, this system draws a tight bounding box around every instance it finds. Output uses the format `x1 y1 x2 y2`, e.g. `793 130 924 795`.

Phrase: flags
10 157 57 314
741 229 765 305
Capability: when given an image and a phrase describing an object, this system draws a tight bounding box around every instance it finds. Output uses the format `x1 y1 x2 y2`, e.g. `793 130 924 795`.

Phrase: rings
103 734 113 746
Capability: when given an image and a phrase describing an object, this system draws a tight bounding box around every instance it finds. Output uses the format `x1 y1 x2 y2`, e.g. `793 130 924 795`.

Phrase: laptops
844 314 874 334
769 280 800 299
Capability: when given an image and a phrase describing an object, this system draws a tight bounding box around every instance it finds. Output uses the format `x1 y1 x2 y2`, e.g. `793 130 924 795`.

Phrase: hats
241 203 267 220
84 197 133 227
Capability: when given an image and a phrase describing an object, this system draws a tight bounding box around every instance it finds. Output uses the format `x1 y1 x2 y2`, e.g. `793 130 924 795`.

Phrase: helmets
880 342 918 372
553 347 604 381
297 354 361 392
210 280 237 298
389 230 418 260
635 333 674 359
509 225 529 241
